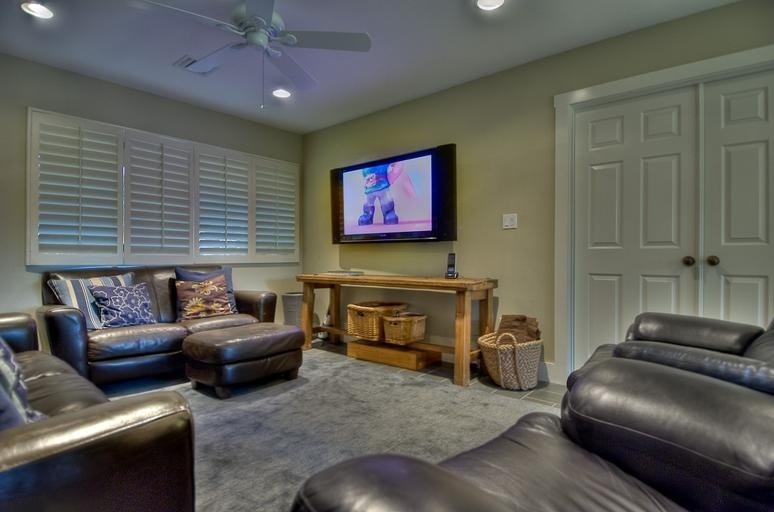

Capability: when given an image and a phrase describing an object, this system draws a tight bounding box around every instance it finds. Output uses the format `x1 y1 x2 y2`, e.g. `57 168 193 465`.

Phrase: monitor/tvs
330 143 457 243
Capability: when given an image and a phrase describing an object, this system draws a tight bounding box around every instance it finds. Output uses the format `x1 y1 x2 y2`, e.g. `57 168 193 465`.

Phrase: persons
357 160 407 226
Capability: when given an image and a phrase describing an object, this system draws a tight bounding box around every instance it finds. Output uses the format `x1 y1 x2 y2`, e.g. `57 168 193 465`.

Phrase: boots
380 200 398 225
357 203 375 225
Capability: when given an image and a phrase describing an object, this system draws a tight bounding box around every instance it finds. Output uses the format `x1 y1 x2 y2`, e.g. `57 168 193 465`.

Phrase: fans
137 0 372 91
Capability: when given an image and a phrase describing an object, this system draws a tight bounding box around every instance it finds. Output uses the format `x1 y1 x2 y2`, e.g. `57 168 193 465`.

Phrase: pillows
0 334 50 430
88 282 157 328
46 272 135 330
176 266 238 314
173 275 232 321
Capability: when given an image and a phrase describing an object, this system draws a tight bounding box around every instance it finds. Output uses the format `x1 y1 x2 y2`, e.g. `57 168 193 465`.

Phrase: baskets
346 300 408 346
478 330 543 391
382 312 428 346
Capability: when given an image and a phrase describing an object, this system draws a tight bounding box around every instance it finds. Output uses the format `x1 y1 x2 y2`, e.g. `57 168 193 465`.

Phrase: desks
296 273 497 388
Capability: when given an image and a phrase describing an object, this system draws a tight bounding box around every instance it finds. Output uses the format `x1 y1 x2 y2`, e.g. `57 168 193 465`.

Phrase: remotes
448 253 455 273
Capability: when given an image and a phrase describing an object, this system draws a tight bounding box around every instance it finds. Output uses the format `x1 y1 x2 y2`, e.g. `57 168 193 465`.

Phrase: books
328 268 365 278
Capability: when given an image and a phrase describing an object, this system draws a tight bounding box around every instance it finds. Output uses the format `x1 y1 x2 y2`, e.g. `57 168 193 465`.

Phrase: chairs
563 312 774 413
292 359 774 512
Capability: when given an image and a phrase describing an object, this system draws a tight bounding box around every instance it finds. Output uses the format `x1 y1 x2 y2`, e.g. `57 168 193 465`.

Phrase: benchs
183 322 305 400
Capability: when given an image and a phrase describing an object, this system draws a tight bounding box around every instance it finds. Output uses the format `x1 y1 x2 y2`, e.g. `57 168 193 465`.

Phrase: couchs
38 265 278 392
0 313 195 512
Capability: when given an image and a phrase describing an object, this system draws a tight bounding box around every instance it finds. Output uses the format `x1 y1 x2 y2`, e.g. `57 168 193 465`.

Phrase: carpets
103 348 563 512
